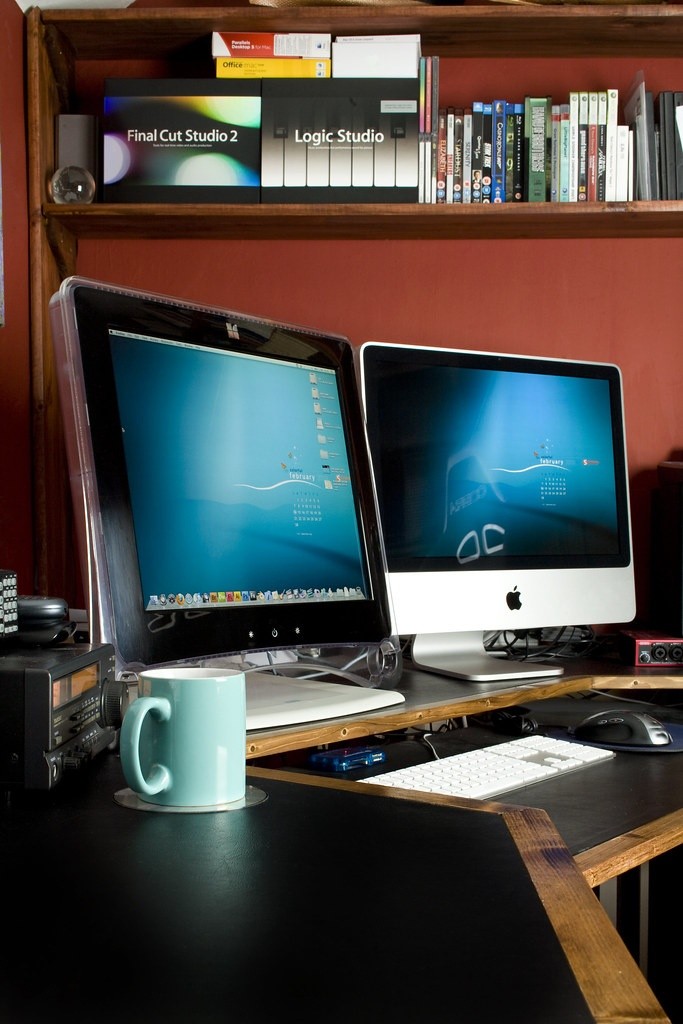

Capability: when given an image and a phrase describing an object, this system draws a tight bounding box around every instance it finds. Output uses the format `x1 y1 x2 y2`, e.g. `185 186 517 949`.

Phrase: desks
0 748 675 1024
243 634 683 889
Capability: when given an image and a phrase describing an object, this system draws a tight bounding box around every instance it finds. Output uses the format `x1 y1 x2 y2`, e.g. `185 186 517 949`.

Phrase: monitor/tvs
359 339 637 682
50 275 407 729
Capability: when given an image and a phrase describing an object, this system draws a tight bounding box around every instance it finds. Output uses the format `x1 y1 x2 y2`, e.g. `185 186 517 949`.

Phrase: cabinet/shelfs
24 4 683 602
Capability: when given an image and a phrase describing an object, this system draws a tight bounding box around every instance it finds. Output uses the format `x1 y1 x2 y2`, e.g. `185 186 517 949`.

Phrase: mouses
573 710 674 748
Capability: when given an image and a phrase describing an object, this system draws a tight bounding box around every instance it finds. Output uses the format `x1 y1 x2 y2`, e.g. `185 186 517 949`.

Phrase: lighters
311 744 387 775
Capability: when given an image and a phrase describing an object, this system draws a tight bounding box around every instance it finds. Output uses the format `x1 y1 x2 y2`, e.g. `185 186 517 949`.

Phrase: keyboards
355 733 616 800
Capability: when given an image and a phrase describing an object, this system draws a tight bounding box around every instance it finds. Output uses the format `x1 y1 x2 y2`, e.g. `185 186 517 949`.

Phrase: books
420 54 683 205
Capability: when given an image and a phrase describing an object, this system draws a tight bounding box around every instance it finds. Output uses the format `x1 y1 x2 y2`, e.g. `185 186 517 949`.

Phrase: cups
120 667 247 807
